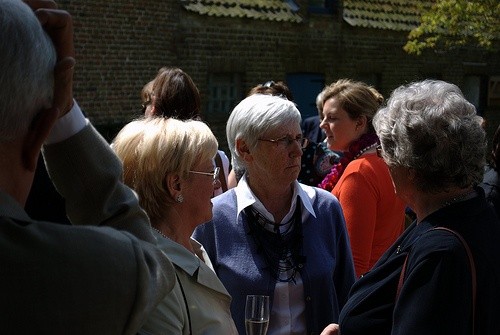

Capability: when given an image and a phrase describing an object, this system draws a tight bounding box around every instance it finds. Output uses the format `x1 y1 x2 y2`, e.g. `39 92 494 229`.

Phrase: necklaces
151 226 176 241
317 133 381 192
242 195 306 284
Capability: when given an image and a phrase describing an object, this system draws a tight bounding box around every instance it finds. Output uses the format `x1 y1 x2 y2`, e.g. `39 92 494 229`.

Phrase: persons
227 79 294 190
321 79 500 335
300 91 343 187
316 80 405 280
108 116 240 335
191 94 357 334
140 65 230 201
0 0 177 335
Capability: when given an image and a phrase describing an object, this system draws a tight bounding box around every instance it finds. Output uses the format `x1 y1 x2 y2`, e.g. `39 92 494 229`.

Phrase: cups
245 295 269 335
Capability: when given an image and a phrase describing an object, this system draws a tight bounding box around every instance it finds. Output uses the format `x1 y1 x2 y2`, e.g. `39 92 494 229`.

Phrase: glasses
140 100 149 113
258 137 310 150
189 167 220 180
376 145 383 159
